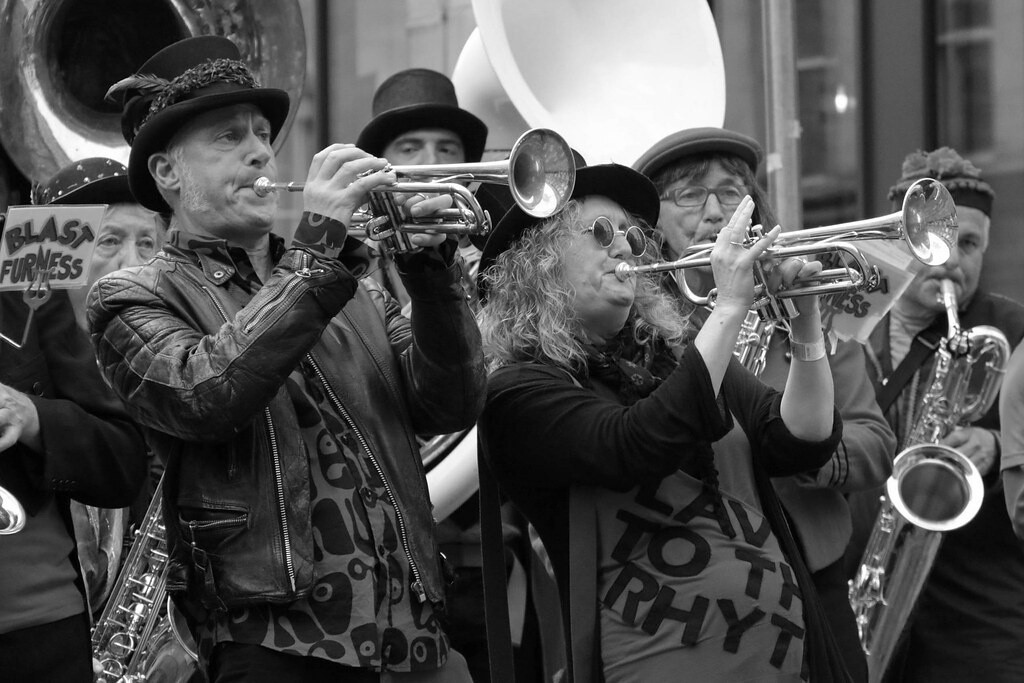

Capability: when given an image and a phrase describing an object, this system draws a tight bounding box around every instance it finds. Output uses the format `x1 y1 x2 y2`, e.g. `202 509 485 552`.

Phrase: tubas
389 0 729 538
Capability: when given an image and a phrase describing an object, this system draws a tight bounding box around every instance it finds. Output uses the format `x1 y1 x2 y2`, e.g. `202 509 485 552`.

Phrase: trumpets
255 126 577 256
620 178 960 348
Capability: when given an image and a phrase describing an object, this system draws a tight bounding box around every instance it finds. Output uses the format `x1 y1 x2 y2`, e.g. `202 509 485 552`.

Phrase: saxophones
841 274 1011 683
92 454 212 683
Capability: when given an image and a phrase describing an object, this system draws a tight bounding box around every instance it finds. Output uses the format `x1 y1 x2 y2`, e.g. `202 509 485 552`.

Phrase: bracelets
788 333 826 362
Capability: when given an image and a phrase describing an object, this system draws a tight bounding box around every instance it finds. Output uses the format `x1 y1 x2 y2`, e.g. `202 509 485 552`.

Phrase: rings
730 241 743 246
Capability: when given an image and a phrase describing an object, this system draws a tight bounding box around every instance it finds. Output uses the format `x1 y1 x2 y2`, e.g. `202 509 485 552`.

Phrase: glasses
579 216 648 257
659 185 755 207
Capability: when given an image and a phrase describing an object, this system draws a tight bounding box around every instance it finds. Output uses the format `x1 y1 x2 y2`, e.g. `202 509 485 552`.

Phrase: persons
1 69 1024 683
472 142 843 683
85 32 488 683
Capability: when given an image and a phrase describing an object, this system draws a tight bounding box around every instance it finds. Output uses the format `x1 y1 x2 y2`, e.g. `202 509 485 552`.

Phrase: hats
475 145 660 305
888 147 995 218
630 127 763 177
106 33 290 213
30 157 127 205
354 68 487 187
